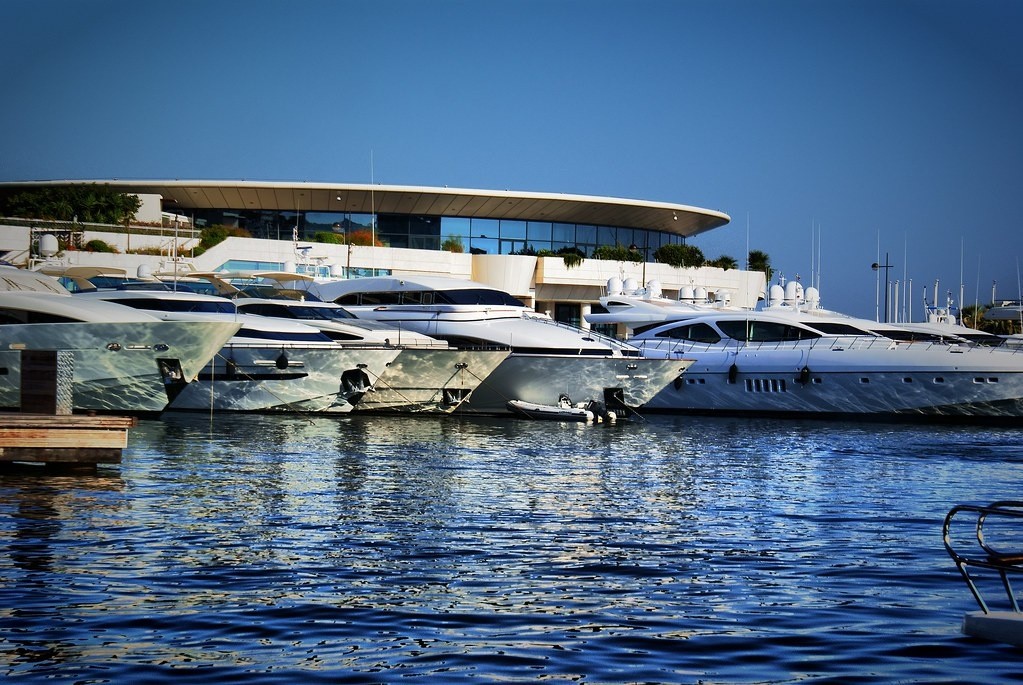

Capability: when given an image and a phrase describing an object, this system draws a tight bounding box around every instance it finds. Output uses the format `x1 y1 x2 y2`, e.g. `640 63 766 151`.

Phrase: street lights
871 253 894 323
331 209 352 280
628 243 652 292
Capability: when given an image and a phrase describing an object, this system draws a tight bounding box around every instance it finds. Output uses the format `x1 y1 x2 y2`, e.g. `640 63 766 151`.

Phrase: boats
1 235 1022 430
506 399 617 426
